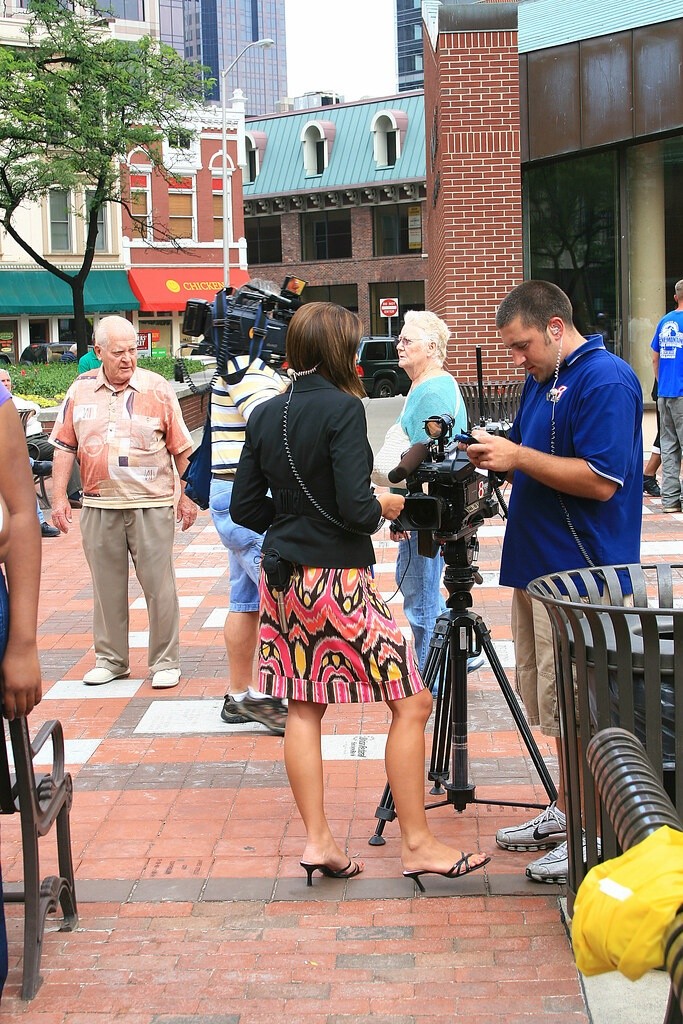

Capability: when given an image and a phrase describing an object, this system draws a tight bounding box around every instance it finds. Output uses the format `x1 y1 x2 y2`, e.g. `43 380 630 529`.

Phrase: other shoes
66 498 83 509
152 668 182 688
41 522 60 537
662 502 681 513
83 666 131 684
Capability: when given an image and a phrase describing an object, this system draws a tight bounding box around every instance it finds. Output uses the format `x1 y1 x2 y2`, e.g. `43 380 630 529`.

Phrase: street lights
221 38 274 288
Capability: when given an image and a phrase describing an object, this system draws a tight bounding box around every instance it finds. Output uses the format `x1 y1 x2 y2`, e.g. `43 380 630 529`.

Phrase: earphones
550 326 558 335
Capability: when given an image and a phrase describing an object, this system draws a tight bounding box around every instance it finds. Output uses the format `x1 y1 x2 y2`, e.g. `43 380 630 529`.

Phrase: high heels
402 849 492 893
299 854 364 887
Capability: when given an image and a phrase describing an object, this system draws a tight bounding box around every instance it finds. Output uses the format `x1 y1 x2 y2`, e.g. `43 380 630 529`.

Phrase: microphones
396 439 436 479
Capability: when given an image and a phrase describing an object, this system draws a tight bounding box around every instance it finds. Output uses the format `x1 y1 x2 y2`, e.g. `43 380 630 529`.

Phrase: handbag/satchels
370 419 457 497
180 399 211 510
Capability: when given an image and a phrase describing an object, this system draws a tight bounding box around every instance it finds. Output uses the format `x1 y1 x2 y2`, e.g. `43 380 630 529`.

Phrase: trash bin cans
522 561 683 920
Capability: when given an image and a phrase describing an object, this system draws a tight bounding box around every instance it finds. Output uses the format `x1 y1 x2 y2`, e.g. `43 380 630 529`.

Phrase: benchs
19 409 80 509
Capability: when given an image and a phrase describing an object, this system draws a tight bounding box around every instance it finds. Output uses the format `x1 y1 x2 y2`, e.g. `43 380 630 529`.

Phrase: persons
227 301 491 893
456 279 644 885
208 277 289 734
385 311 485 701
0 385 44 981
45 314 197 689
644 279 683 514
0 332 102 537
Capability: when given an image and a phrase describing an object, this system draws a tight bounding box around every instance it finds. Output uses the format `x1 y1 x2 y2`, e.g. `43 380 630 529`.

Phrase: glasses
395 336 422 345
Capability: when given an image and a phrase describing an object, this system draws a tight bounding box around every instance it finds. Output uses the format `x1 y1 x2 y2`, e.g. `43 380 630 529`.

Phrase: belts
213 471 236 481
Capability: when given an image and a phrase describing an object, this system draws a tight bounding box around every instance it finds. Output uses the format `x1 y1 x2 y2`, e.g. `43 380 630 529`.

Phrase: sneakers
427 677 452 700
642 474 661 497
495 800 585 852
524 829 602 884
433 657 484 682
220 694 257 724
240 691 288 732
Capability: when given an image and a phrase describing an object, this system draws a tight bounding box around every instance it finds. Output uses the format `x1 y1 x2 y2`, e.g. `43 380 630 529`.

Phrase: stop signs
379 298 399 318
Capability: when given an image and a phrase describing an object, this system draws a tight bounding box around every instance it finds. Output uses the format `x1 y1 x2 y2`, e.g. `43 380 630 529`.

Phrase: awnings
129 269 252 312
0 271 140 316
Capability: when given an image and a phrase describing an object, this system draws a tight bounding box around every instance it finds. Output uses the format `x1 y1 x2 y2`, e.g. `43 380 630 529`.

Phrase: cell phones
455 434 479 445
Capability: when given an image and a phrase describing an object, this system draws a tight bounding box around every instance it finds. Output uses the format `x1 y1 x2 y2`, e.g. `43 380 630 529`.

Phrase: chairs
0 716 78 1000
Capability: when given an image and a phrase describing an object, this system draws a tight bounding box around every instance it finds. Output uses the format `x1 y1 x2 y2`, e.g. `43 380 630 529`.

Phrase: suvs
355 333 412 399
20 342 94 364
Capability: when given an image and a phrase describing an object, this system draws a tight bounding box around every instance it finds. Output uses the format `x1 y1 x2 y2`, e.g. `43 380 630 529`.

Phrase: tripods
368 526 560 845
